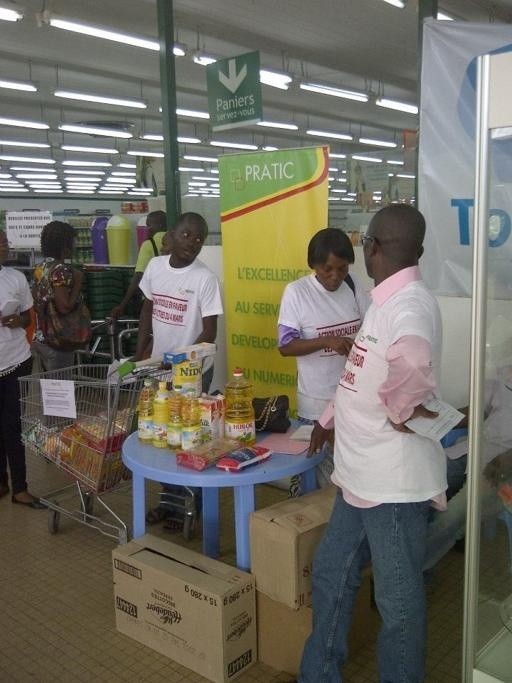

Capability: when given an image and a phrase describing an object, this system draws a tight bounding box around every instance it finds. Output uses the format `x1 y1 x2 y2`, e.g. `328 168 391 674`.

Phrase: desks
120 416 330 574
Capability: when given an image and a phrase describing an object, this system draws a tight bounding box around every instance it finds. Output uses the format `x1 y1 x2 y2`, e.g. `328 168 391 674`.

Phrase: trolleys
19 360 199 545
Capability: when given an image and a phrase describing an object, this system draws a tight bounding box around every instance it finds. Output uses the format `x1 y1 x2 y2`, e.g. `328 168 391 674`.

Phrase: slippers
161 516 185 532
146 509 163 525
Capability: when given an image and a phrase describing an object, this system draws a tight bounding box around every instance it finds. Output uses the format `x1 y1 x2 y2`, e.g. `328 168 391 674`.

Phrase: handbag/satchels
41 263 93 349
250 394 292 434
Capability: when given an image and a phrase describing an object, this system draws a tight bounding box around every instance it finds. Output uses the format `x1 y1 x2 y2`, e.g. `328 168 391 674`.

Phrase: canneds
172 361 202 397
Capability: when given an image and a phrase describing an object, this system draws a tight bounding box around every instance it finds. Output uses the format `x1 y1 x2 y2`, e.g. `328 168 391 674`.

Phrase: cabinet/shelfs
68 215 95 266
0 248 36 290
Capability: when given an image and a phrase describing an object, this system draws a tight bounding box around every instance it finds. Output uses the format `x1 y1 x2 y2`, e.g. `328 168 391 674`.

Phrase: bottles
166 386 190 448
224 368 256 447
211 389 225 439
164 342 217 364
181 389 205 450
138 379 157 445
198 393 216 441
152 382 169 448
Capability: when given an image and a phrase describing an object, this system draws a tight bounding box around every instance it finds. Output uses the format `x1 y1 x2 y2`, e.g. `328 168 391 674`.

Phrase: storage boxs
252 588 316 677
247 486 341 609
111 532 258 682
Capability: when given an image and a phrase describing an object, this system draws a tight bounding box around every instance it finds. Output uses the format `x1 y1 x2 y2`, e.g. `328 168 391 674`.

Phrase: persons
0 229 49 511
128 212 224 535
29 221 92 380
298 204 447 683
277 228 362 491
112 211 167 317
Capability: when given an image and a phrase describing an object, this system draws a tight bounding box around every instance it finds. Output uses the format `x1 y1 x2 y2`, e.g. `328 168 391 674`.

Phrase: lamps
137 129 206 145
158 101 211 121
191 48 294 92
255 120 299 131
55 119 133 139
358 137 397 148
298 78 377 102
0 76 40 92
0 114 51 131
206 139 279 152
0 7 25 23
34 8 188 57
303 127 354 141
374 96 420 115
383 0 407 10
48 85 148 109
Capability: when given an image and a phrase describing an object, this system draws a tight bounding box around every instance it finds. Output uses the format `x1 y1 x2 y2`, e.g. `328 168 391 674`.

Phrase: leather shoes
11 493 48 510
